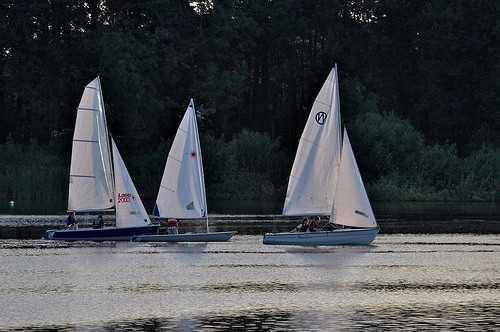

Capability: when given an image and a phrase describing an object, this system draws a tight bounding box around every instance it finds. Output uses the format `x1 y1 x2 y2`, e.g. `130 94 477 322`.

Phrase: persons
166 218 179 235
65 212 79 230
290 218 310 232
309 216 321 232
93 215 104 229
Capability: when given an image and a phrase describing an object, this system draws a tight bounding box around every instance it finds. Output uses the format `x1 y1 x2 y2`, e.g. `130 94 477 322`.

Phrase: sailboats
263 63 380 246
132 98 237 242
45 75 161 241
